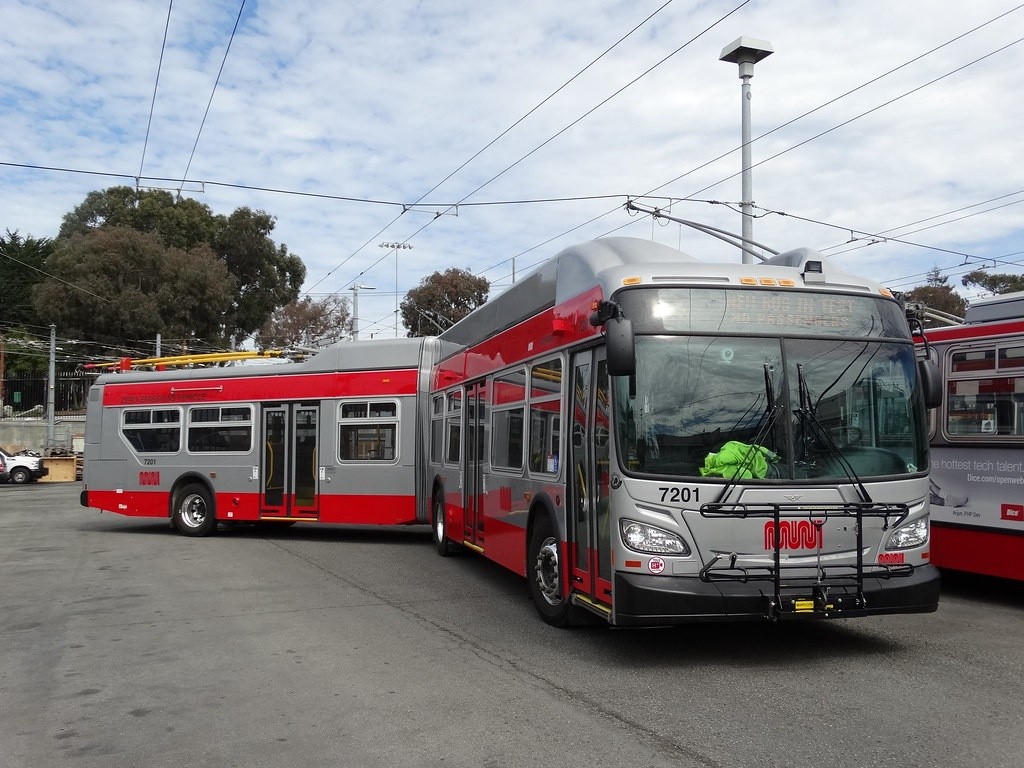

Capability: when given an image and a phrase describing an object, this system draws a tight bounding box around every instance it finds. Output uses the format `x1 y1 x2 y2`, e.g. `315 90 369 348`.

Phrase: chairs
122 426 316 487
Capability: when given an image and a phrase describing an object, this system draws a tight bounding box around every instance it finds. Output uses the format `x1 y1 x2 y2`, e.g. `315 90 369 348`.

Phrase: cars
0 447 49 484
910 318 1024 579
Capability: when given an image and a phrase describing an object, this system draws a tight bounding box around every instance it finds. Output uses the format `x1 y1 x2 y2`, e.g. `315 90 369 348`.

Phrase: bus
427 237 941 626
80 335 439 538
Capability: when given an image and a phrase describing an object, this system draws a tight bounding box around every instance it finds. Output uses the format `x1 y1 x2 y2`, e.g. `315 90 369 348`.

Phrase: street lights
720 36 774 265
379 242 414 337
349 284 377 341
